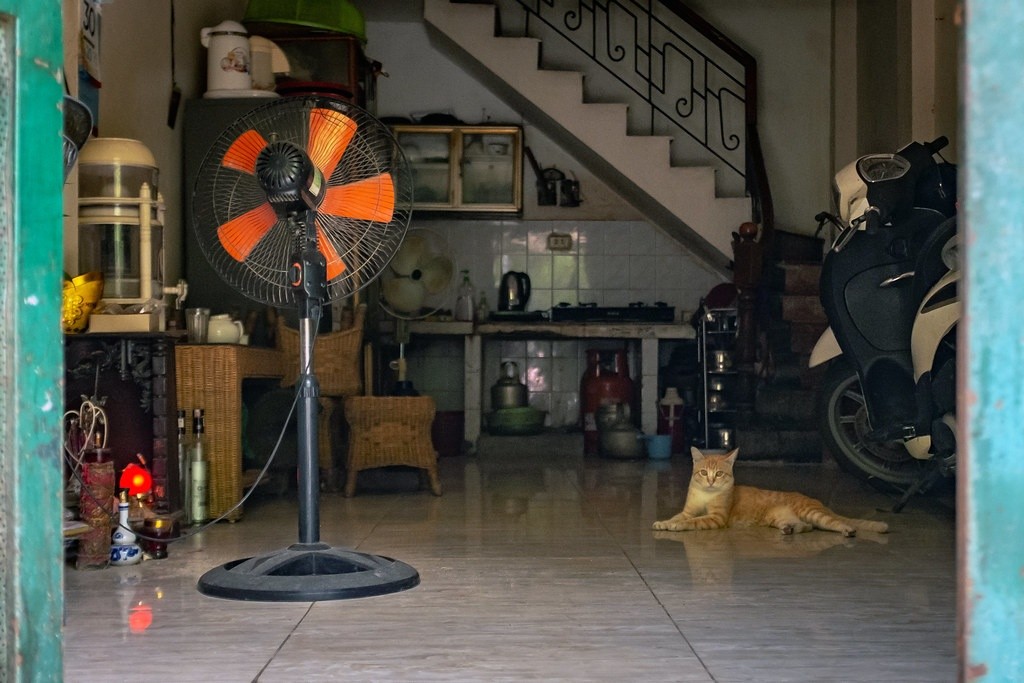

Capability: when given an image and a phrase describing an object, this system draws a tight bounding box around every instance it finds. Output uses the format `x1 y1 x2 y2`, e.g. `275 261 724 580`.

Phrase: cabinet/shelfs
391 123 525 221
267 34 358 109
698 308 737 450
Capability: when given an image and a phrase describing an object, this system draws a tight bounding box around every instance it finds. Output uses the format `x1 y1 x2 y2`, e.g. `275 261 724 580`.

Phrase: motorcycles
807 135 957 512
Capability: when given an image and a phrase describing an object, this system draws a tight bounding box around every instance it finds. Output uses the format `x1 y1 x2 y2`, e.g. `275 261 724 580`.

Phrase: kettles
490 361 527 408
201 21 281 97
598 417 644 458
498 270 530 311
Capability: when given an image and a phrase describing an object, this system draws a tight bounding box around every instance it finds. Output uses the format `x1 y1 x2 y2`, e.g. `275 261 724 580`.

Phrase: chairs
342 393 443 496
277 303 367 494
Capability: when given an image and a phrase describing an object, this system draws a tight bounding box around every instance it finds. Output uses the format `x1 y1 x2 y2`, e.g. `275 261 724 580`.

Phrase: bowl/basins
488 144 509 156
483 409 548 434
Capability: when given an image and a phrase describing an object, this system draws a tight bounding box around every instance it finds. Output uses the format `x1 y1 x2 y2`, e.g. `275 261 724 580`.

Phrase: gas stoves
552 301 675 323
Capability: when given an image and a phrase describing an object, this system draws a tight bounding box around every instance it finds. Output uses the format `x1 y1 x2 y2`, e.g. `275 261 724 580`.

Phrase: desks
176 345 332 523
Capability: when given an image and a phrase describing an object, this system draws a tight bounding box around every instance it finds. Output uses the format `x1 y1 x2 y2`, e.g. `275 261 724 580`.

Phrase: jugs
660 387 686 452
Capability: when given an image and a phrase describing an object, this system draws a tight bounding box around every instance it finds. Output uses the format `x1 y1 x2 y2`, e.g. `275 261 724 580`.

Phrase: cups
184 307 210 343
146 518 173 559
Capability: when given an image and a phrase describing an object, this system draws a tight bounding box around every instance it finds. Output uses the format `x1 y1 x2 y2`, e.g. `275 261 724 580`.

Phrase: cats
650 444 889 537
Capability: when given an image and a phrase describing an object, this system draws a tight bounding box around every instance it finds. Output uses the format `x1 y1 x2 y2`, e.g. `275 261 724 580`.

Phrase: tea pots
207 315 243 344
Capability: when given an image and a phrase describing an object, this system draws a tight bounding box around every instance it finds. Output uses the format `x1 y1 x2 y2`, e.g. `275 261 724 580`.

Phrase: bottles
476 298 490 323
186 409 209 524
112 503 142 565
176 408 200 521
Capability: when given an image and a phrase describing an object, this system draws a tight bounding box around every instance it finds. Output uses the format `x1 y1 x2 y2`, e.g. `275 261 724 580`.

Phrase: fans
373 225 461 472
190 91 420 604
